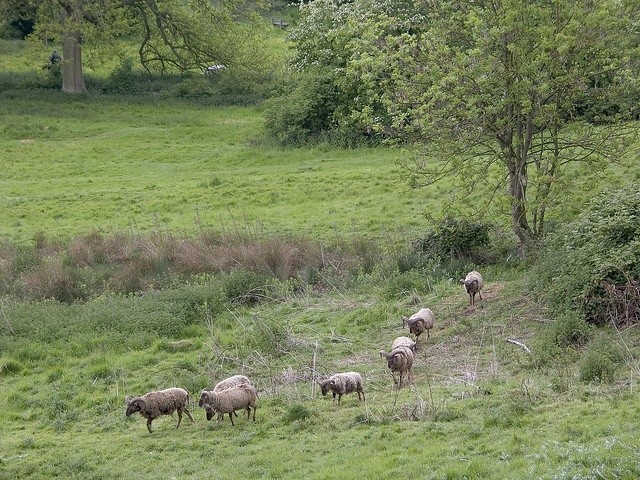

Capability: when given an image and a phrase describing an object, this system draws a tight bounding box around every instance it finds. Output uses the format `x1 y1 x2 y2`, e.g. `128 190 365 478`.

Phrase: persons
49 51 62 67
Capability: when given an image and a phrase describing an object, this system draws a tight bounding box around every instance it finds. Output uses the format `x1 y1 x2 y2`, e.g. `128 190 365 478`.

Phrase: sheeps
459 270 485 306
205 373 251 421
390 335 417 354
198 382 262 427
125 387 196 434
379 346 415 389
316 371 366 407
401 307 435 343
